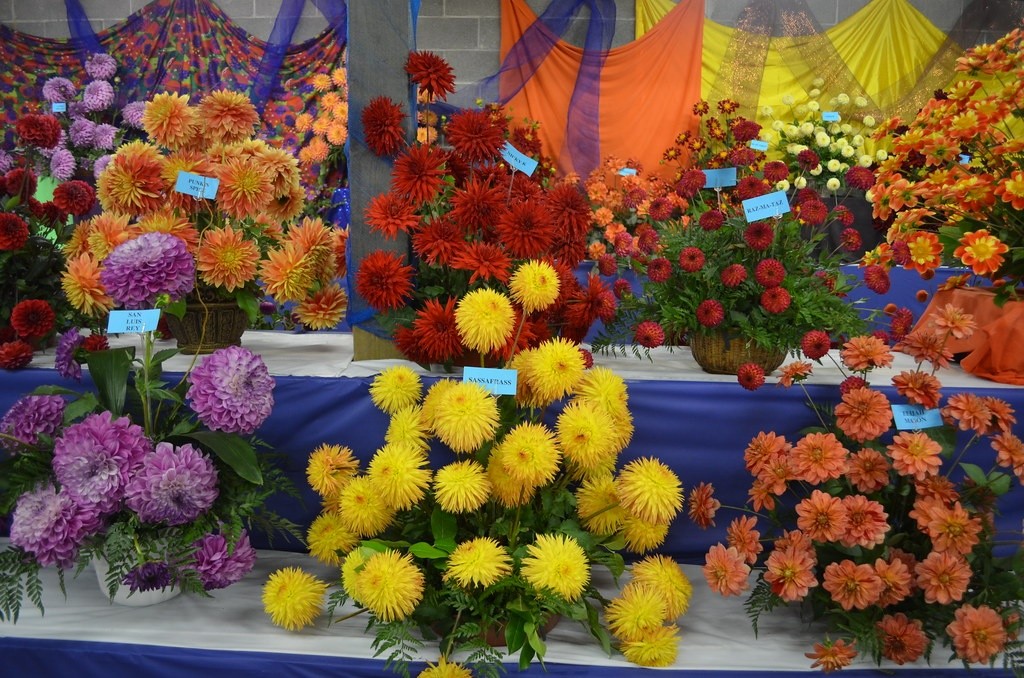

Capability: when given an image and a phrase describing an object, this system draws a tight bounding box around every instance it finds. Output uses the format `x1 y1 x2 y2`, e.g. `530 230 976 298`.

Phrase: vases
162 303 248 355
419 585 561 646
702 320 790 376
90 528 182 605
787 188 901 261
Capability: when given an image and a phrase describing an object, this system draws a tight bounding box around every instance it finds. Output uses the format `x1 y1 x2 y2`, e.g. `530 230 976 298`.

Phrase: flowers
0 232 280 628
0 9 1024 371
264 256 693 677
688 336 1024 677
